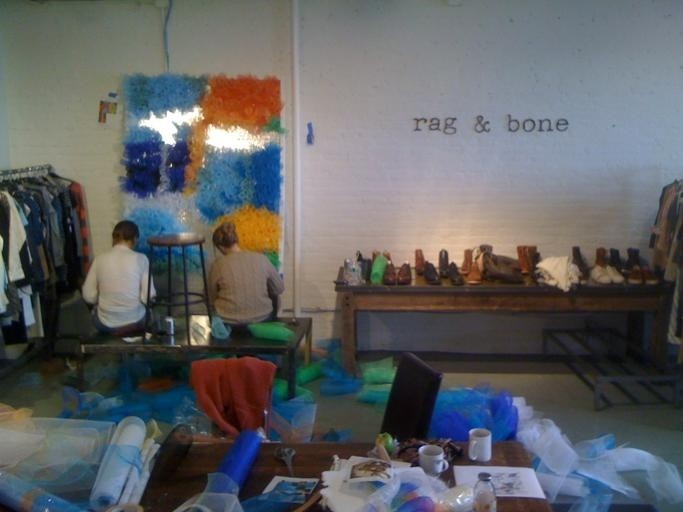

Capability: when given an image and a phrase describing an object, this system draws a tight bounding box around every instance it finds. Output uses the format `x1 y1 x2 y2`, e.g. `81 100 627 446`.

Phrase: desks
333 267 671 375
76 315 312 399
137 441 549 512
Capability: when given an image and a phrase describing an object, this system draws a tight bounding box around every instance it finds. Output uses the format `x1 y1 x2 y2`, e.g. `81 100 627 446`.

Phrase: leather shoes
355 244 540 286
572 245 659 285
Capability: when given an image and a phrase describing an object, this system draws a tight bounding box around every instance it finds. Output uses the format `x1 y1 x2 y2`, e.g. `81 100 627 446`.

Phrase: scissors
272 445 296 477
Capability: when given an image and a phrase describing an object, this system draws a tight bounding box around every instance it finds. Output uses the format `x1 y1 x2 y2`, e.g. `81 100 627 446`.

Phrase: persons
202 221 285 332
80 219 156 340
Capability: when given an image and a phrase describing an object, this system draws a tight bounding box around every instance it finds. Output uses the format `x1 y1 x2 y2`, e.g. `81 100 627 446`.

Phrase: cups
418 444 449 474
467 428 492 463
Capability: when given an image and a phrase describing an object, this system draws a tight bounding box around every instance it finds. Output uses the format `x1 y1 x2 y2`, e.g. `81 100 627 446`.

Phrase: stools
144 232 212 328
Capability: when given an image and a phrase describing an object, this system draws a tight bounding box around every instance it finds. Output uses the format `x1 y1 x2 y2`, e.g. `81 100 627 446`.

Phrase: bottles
472 472 497 512
